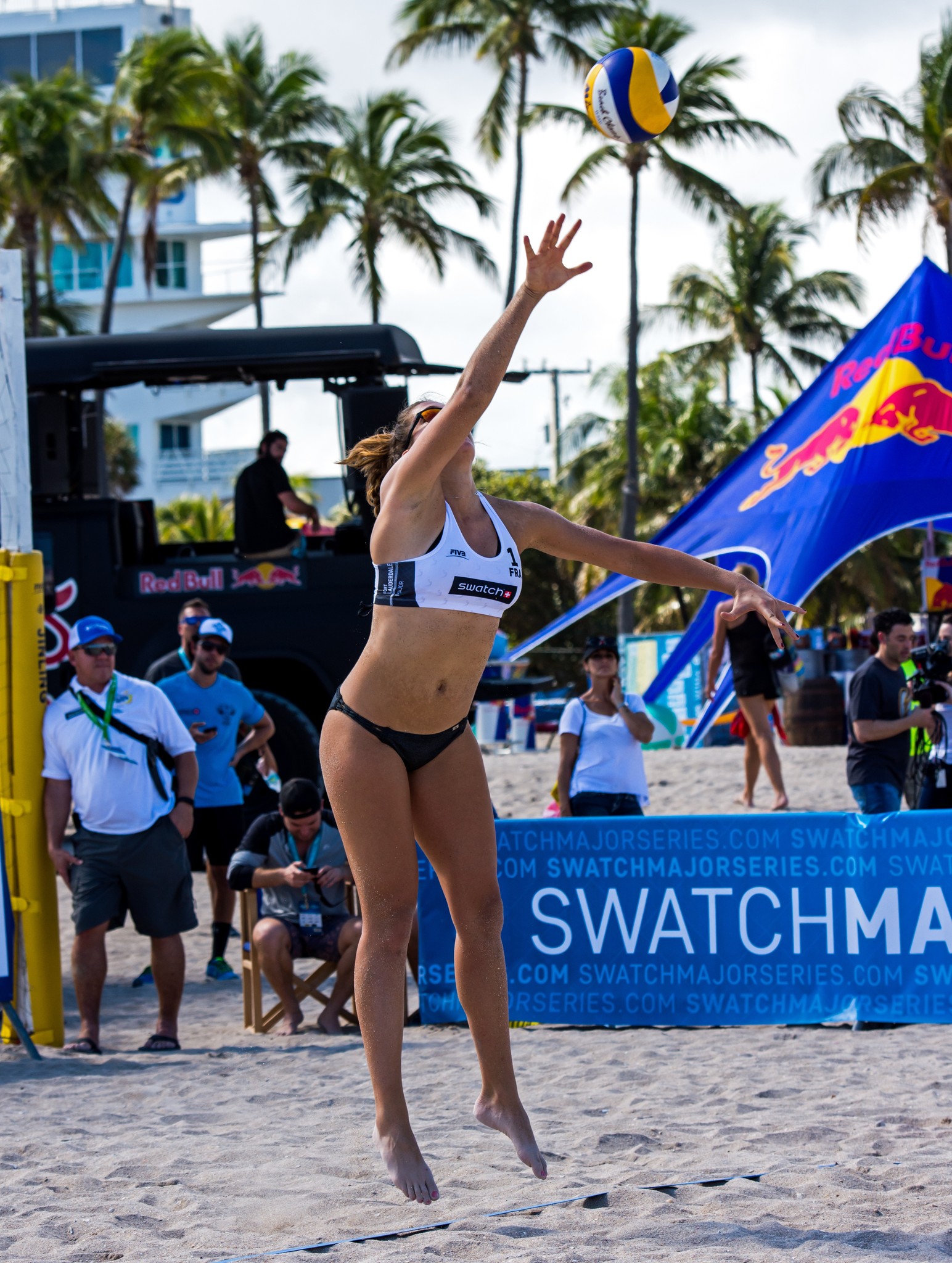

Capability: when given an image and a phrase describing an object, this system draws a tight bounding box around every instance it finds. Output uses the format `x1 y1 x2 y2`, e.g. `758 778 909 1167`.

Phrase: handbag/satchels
773 651 799 695
769 643 805 677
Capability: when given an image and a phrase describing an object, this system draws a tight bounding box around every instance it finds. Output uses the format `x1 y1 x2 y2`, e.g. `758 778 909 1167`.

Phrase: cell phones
203 726 217 733
303 866 319 875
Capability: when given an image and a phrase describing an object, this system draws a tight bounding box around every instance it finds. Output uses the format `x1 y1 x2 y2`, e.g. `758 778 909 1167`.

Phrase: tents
508 254 952 748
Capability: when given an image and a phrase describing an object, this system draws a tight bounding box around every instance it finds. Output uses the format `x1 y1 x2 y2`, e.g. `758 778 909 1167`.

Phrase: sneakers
204 955 239 982
132 964 155 986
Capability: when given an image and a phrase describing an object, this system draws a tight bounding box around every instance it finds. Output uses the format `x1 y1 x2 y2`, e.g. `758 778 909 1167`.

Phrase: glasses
179 616 210 625
404 406 475 450
590 653 614 661
198 640 227 655
81 644 116 657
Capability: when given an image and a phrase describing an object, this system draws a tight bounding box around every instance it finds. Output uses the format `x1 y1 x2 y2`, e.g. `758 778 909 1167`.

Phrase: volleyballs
581 45 681 144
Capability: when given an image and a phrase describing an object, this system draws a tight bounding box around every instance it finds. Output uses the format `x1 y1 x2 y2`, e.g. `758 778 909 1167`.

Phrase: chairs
237 879 362 1036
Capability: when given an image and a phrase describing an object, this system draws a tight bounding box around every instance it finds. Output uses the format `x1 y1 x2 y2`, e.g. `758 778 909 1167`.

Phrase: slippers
64 1037 107 1056
138 1034 181 1051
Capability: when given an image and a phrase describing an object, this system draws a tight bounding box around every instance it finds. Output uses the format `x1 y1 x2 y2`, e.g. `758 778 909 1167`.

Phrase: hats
198 618 234 645
584 634 619 660
278 778 320 819
68 616 122 650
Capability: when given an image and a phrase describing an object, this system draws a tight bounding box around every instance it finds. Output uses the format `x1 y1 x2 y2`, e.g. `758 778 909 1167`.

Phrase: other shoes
227 925 242 938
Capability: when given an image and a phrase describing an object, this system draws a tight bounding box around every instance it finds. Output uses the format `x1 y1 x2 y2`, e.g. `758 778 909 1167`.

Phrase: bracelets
616 703 626 710
176 795 195 806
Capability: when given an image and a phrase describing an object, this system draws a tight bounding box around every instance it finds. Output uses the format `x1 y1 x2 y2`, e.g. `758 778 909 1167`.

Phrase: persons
135 598 279 988
232 430 321 565
227 778 362 1036
317 216 808 1207
555 564 952 817
41 617 197 1055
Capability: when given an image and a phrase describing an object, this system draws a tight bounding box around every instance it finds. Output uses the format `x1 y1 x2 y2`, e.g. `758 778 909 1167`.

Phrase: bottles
255 757 282 793
